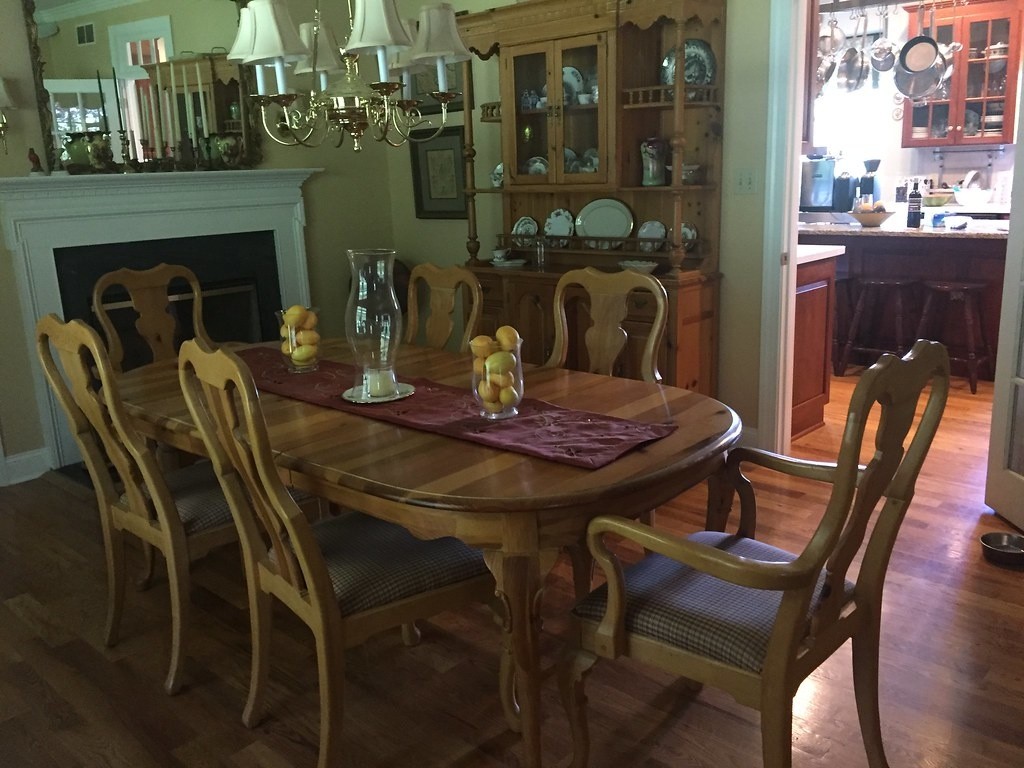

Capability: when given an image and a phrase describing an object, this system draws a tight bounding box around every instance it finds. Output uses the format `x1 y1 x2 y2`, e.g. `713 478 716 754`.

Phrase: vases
62 132 91 167
344 247 401 398
197 137 221 162
84 132 114 168
217 132 243 168
640 137 666 185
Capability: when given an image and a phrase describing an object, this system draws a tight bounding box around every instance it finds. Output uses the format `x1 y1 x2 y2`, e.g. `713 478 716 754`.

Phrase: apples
859 201 883 211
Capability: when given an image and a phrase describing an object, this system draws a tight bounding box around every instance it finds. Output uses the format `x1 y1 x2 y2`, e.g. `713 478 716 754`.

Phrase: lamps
228 0 478 152
0 73 17 156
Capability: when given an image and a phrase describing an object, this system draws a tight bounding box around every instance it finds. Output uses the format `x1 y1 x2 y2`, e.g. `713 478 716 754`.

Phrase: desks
100 341 742 768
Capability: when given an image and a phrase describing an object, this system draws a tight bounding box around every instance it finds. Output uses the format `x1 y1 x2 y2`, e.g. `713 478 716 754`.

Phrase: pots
816 4 946 107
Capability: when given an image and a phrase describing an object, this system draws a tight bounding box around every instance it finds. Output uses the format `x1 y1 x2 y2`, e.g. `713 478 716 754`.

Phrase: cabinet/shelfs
453 0 728 400
141 53 256 160
790 258 837 443
799 236 1007 383
903 0 1024 146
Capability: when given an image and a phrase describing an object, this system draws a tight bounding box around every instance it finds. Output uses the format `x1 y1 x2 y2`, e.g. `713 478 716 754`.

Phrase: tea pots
489 161 504 188
591 152 599 173
981 41 1008 55
579 163 598 173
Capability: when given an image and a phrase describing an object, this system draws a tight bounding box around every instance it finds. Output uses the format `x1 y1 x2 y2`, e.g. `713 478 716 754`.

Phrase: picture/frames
410 9 474 118
410 126 467 220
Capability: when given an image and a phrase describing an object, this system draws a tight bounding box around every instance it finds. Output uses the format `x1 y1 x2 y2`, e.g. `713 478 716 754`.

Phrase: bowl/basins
847 211 896 227
923 195 953 207
979 531 1024 568
619 261 658 277
953 189 993 206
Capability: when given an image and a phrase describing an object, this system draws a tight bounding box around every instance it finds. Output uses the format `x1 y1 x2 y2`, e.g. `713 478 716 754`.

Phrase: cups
578 94 592 105
540 97 547 108
275 305 324 374
468 336 524 421
969 48 979 59
491 248 511 261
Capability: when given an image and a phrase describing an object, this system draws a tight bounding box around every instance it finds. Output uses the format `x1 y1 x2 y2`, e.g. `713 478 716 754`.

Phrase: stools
914 279 992 395
839 276 917 377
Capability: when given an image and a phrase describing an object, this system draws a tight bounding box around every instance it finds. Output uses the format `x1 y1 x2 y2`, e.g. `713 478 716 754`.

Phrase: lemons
469 325 521 413
280 305 319 362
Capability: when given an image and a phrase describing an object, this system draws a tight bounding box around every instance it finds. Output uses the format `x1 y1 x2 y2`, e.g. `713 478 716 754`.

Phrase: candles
97 70 109 131
182 66 195 138
119 86 129 140
166 92 174 147
112 67 124 131
145 95 154 148
189 93 198 147
141 87 148 141
196 63 208 137
149 86 163 159
169 62 183 141
156 64 166 143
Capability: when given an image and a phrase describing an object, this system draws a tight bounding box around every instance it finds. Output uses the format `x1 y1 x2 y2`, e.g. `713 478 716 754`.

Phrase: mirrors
22 0 261 174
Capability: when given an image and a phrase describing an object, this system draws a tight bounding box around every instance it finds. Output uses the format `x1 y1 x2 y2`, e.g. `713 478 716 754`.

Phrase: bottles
906 178 922 227
345 247 403 400
640 136 665 185
520 89 538 111
63 101 244 170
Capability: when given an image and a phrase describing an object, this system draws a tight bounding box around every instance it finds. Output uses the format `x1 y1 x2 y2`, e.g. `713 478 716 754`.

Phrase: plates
489 38 717 267
965 102 1004 137
912 126 929 138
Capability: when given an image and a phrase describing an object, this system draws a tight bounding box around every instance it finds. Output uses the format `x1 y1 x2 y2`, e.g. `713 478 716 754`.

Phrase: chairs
32 315 330 697
175 338 521 768
541 267 666 603
559 332 950 768
93 264 213 378
411 260 484 355
